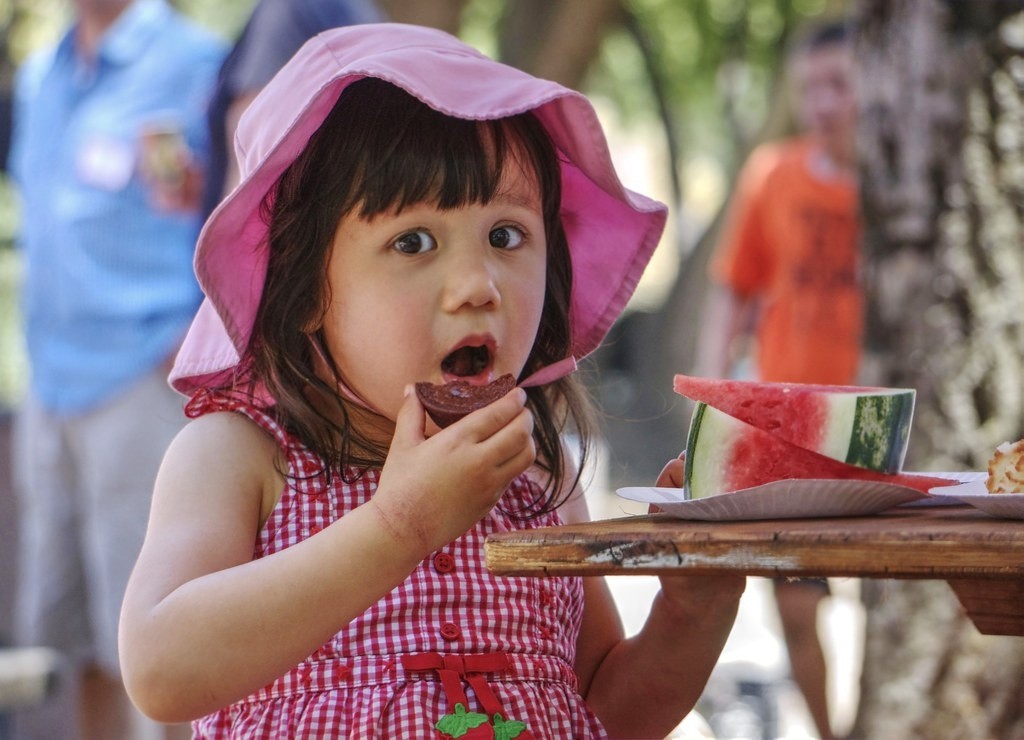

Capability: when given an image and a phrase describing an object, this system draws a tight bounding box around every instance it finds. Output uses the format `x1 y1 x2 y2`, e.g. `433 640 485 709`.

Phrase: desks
484 502 1024 637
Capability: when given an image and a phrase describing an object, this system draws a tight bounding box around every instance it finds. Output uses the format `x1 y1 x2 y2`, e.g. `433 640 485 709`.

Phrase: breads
986 439 1024 493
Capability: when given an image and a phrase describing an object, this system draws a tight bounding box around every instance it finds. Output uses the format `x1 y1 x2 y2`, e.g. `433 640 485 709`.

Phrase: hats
166 21 670 407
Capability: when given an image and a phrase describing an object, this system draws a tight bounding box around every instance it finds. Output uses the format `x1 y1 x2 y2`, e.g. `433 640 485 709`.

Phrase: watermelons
671 373 957 500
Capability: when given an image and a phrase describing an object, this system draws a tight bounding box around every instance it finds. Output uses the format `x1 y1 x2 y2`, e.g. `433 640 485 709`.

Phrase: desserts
415 373 515 430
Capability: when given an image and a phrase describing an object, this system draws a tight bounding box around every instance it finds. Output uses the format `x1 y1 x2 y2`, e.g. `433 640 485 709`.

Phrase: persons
688 17 869 739
131 0 387 255
112 19 757 740
7 0 228 739
